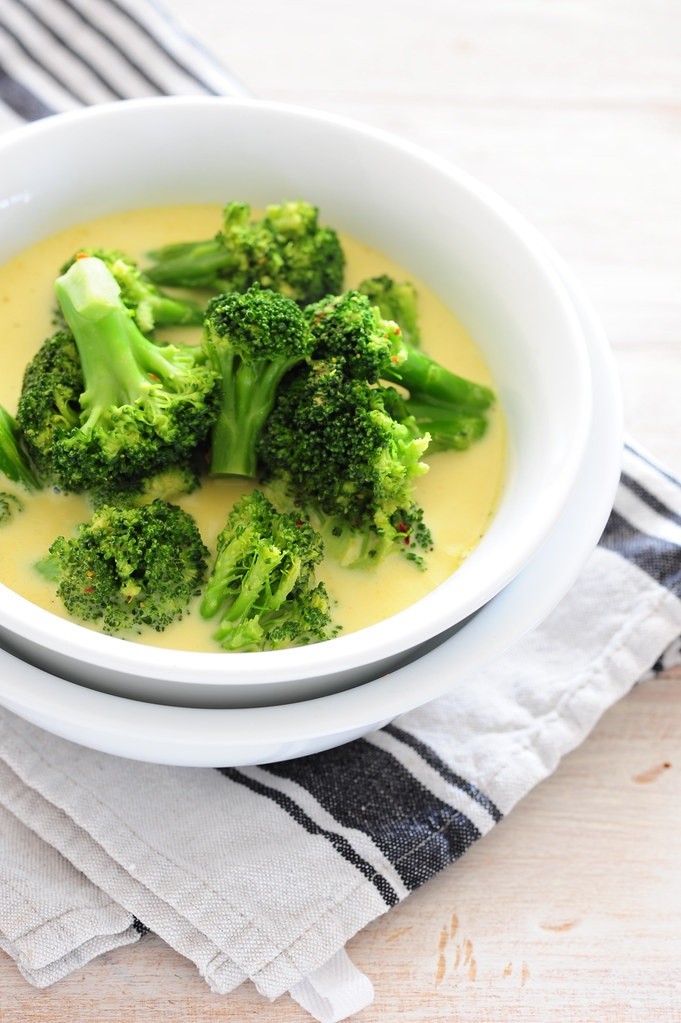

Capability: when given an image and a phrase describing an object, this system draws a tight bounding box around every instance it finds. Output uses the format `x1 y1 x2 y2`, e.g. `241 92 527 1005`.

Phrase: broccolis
0 200 495 653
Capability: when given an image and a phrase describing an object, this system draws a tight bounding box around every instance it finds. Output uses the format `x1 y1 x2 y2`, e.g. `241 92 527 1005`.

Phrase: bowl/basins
0 93 625 768
